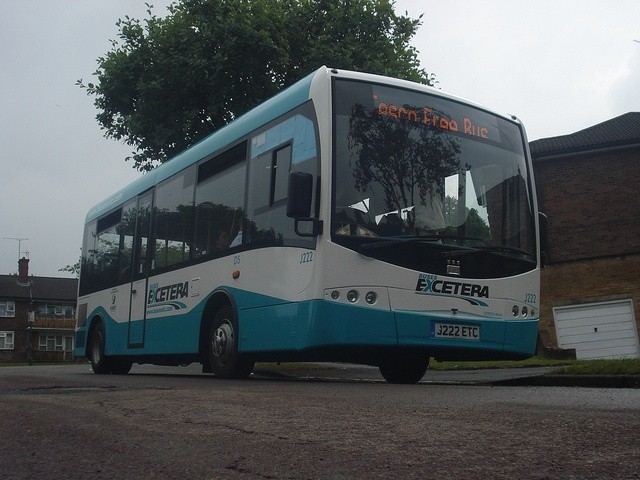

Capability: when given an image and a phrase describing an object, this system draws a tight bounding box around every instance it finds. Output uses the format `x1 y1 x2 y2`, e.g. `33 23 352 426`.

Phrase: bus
74 64 548 384
